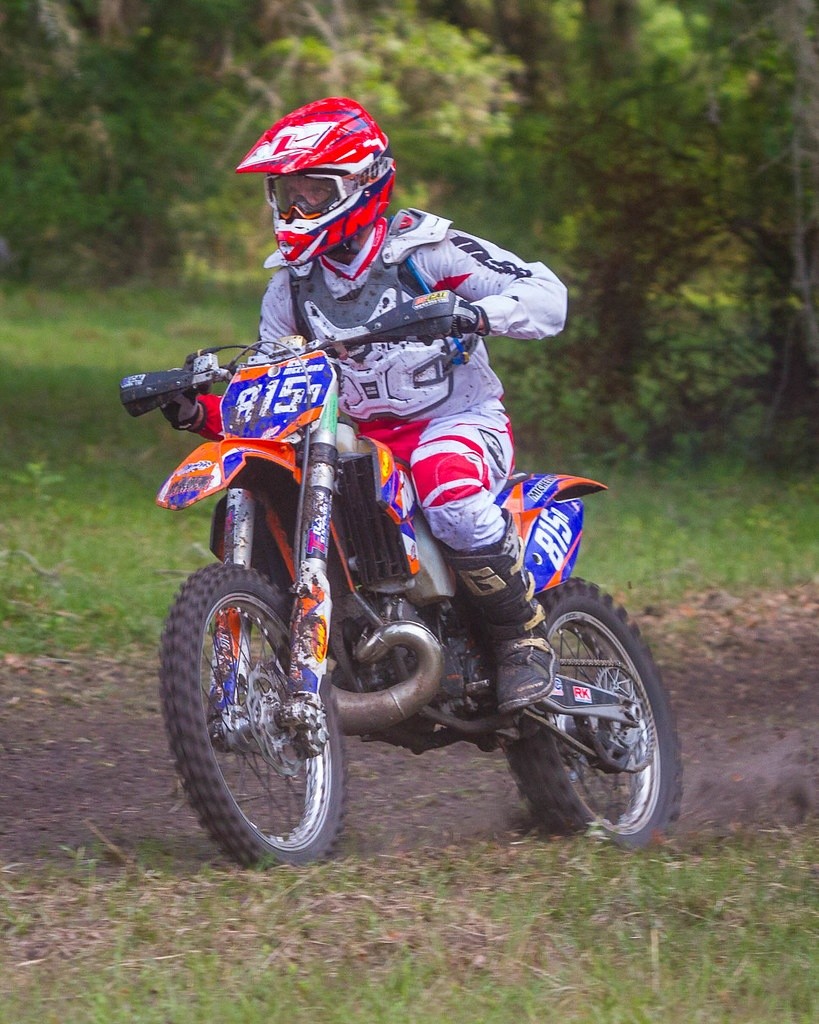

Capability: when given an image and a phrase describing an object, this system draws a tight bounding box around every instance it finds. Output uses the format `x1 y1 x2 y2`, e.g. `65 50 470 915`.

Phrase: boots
441 508 560 714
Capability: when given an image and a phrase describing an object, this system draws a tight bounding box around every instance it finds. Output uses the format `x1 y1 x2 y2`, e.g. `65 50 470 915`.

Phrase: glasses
272 175 340 214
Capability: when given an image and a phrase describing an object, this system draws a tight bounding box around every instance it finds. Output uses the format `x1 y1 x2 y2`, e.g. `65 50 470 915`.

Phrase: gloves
416 294 481 346
159 367 199 430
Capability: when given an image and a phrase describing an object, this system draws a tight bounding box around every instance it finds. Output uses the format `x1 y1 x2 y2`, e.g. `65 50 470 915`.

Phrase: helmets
235 96 397 266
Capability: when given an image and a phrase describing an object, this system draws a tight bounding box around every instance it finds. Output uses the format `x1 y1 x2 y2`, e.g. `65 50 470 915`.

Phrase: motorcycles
119 291 684 873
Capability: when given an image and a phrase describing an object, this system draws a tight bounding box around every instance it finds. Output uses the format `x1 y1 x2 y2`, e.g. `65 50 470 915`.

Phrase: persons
158 97 568 715
